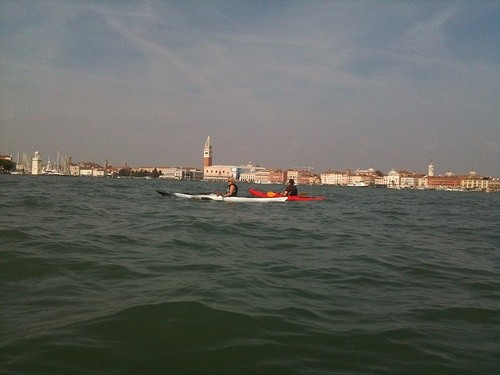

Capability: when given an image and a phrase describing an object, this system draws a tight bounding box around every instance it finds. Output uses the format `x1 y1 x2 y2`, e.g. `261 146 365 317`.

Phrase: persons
215 177 238 198
280 178 299 197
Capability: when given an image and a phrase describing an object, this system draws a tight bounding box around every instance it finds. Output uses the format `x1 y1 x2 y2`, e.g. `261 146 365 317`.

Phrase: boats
158 190 289 203
249 188 326 201
